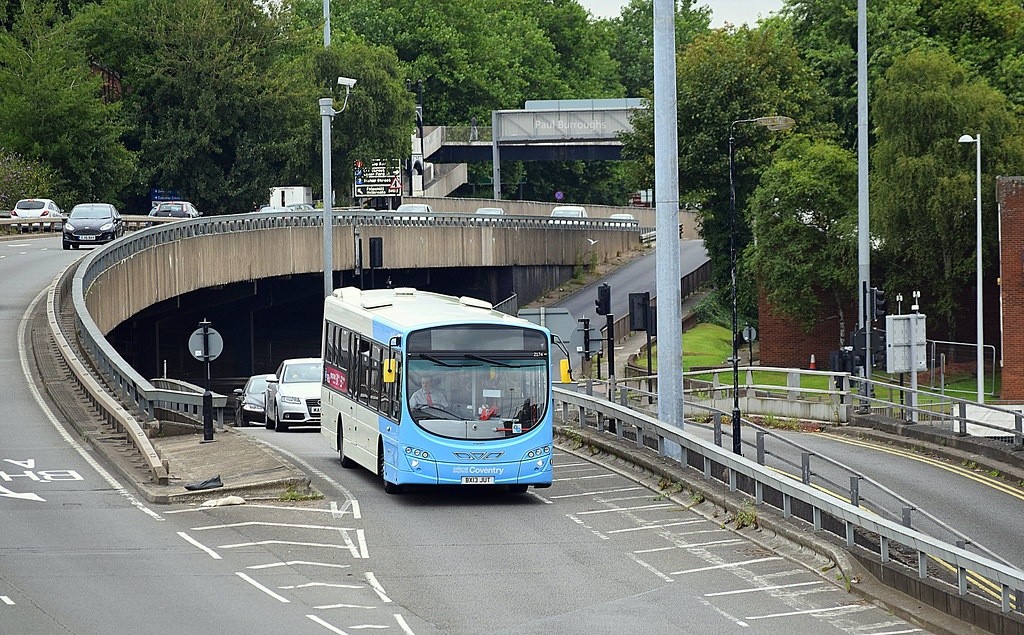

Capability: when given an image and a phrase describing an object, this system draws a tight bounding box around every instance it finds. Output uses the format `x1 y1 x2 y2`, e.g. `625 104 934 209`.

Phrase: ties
426 391 432 408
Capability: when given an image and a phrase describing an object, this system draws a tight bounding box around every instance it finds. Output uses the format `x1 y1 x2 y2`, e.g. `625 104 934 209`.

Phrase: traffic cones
807 353 818 369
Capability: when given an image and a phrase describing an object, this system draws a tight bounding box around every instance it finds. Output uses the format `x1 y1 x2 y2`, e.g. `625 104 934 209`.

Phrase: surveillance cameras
338 77 358 88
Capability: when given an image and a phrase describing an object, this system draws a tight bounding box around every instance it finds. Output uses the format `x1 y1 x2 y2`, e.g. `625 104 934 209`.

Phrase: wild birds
588 239 598 245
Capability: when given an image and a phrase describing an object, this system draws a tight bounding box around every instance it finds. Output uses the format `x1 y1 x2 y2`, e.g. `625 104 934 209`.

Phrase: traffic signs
353 184 401 198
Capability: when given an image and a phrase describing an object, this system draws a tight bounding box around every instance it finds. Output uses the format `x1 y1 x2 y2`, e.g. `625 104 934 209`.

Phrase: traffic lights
595 284 605 316
872 287 885 321
873 335 886 366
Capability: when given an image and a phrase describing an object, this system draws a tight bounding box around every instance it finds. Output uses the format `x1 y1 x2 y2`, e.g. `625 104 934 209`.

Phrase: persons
409 373 448 409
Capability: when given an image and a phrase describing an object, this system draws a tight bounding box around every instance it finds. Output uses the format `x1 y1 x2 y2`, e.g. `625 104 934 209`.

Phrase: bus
319 284 575 498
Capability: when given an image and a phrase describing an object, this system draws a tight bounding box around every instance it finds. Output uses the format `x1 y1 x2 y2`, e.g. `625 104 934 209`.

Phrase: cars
231 374 276 428
262 358 323 432
547 206 588 224
10 198 66 232
61 202 124 249
392 204 435 221
467 207 510 223
603 213 636 227
146 201 204 228
258 203 396 221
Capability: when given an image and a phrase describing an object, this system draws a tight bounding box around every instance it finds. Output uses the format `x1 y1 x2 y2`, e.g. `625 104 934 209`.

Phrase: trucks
269 186 312 209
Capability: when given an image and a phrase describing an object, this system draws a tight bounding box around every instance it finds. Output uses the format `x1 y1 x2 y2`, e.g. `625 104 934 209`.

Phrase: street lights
725 114 797 457
318 76 356 295
956 132 988 404
